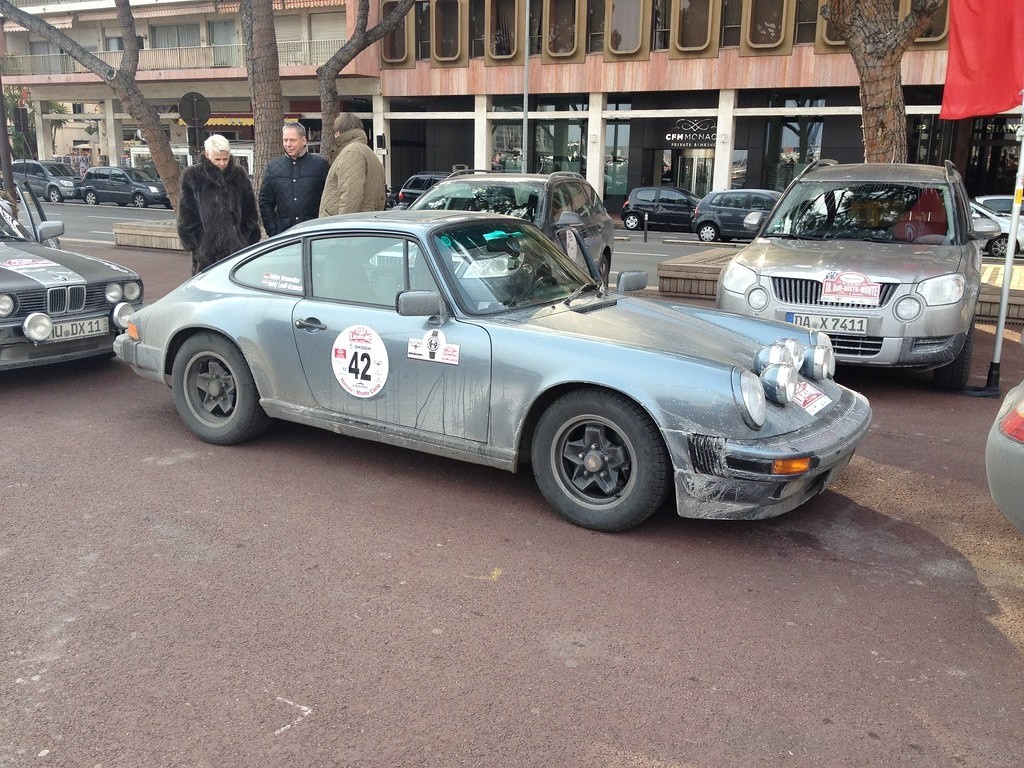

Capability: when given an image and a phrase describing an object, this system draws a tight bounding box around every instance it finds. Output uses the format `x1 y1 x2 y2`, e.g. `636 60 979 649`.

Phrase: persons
498 151 507 173
258 121 331 237
177 134 261 278
318 114 385 218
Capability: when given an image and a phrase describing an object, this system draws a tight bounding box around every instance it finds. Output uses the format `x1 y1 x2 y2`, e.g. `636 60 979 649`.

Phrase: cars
620 186 704 232
984 379 1024 535
976 195 1023 214
0 204 144 371
967 200 1024 258
112 209 872 531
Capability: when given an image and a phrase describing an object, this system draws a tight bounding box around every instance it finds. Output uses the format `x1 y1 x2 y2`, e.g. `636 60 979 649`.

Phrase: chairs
325 247 379 303
751 197 762 209
413 238 454 291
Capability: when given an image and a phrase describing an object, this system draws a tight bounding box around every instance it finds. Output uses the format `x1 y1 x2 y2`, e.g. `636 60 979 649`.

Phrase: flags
893 182 946 246
938 0 1024 119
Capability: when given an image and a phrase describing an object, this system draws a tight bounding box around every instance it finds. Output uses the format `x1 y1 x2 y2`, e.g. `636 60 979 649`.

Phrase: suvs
9 158 83 203
691 188 785 242
407 168 615 312
399 171 455 209
715 159 983 387
79 167 174 210
